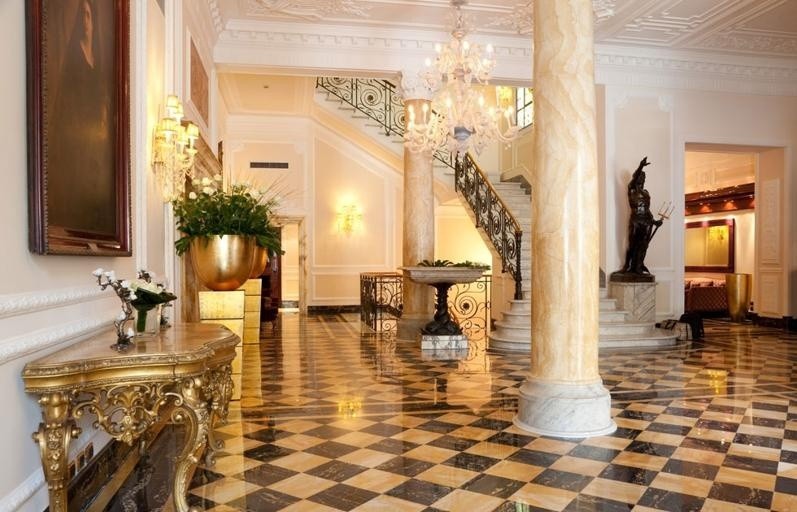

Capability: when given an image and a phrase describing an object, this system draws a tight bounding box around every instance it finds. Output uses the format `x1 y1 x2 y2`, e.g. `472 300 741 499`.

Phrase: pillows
700 282 713 287
689 281 701 287
684 281 690 288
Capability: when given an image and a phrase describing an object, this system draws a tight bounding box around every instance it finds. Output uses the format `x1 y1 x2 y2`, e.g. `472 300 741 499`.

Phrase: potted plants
399 259 491 335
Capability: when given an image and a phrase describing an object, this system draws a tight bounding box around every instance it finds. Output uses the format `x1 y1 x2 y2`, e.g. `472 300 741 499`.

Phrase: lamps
407 35 522 161
335 205 362 236
152 95 180 203
178 123 200 180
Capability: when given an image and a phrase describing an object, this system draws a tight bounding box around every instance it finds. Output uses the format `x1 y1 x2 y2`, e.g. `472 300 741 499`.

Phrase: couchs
684 278 727 317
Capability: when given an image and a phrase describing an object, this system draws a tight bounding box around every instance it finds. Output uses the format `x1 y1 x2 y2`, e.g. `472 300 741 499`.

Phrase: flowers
173 181 280 252
131 281 177 331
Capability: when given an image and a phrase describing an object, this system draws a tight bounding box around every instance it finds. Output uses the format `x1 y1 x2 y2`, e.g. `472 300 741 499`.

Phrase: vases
247 245 267 279
189 234 253 290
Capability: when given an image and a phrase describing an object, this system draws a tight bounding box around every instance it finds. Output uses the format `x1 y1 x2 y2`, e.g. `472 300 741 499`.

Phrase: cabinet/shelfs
21 323 241 512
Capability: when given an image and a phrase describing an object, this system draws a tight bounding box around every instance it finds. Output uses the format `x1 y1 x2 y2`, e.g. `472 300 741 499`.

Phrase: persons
52 0 116 237
621 156 663 274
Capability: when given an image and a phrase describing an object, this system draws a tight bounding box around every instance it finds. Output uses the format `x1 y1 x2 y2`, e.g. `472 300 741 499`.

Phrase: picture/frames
24 0 133 257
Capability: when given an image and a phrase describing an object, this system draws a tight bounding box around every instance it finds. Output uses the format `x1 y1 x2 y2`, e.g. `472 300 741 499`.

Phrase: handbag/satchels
660 321 692 341
